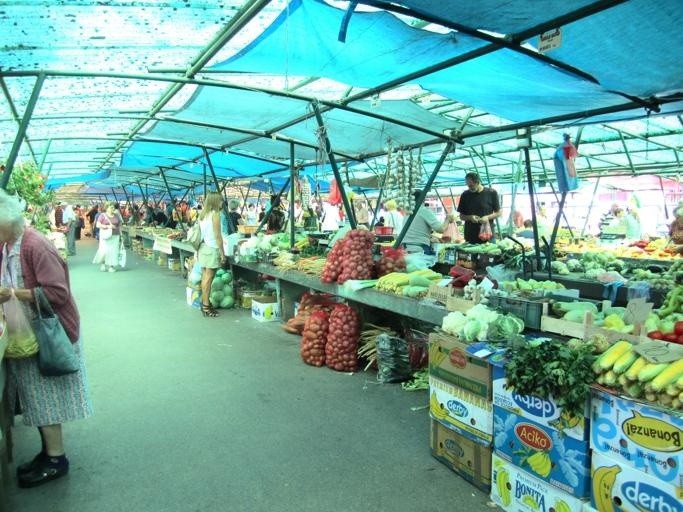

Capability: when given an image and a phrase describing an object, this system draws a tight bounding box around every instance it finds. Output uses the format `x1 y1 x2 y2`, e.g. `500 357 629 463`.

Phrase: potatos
284 293 360 372
321 230 406 284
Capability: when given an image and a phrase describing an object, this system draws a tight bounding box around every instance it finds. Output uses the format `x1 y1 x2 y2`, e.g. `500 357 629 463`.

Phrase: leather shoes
17 453 70 489
15 451 45 477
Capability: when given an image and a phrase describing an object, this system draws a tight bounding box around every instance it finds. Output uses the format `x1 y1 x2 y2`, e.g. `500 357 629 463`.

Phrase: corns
593 341 683 408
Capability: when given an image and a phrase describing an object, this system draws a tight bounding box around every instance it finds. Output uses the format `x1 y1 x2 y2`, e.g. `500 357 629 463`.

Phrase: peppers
658 286 683 317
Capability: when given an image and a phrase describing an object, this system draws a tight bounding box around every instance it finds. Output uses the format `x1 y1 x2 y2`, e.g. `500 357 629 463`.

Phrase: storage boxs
590 450 683 511
429 332 492 402
582 501 597 511
427 373 494 449
429 416 491 494
491 362 589 441
490 450 584 512
589 384 682 488
492 404 590 503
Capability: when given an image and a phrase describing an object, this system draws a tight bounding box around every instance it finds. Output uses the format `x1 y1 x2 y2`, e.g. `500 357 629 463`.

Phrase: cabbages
209 270 234 309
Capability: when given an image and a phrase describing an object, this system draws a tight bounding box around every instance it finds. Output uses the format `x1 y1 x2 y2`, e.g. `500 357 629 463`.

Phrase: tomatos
647 321 683 344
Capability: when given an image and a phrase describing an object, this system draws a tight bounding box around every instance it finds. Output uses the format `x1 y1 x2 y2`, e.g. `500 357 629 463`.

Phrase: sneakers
108 267 117 273
100 263 106 271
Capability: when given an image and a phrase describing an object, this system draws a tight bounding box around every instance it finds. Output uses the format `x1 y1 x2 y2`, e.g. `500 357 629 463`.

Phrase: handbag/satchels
28 287 82 377
186 220 202 252
100 228 113 240
118 240 127 269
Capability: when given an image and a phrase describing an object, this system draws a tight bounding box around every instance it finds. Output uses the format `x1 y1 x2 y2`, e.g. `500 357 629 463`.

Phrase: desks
121 221 674 377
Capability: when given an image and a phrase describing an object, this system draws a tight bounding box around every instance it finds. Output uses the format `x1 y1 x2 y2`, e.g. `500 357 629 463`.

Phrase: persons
46 192 403 318
608 208 625 227
517 220 535 238
0 195 94 490
457 173 503 244
398 190 455 255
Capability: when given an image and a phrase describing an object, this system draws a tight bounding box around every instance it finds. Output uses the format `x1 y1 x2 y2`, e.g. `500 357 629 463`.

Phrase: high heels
202 303 219 318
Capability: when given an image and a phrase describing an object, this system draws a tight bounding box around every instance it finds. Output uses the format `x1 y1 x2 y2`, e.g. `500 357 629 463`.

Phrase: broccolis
441 304 524 342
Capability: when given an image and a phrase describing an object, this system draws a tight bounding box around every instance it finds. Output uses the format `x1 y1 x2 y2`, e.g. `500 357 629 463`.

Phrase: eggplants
553 301 626 323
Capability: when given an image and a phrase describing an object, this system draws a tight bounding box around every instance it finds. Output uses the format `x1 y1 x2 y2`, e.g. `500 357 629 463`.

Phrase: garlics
396 150 417 215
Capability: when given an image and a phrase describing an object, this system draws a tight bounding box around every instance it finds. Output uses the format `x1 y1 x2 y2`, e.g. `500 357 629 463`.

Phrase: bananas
527 451 551 478
430 391 449 420
523 496 538 508
593 465 621 512
496 466 511 505
556 500 571 512
